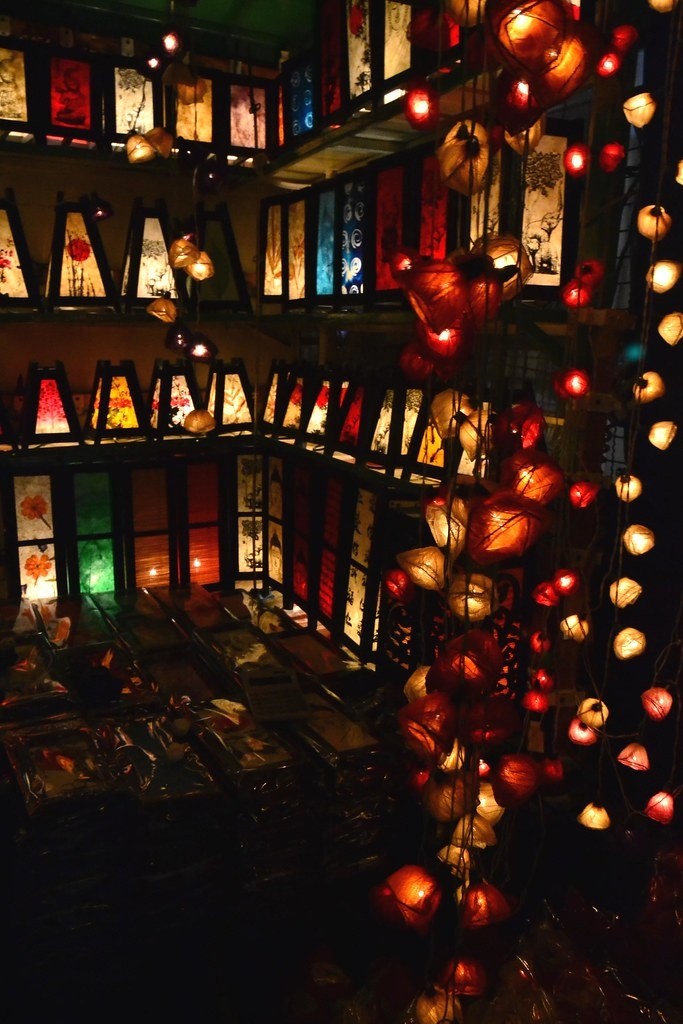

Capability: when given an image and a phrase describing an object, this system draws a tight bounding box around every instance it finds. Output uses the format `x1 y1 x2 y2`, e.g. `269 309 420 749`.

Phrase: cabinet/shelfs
0 17 279 463
257 9 640 515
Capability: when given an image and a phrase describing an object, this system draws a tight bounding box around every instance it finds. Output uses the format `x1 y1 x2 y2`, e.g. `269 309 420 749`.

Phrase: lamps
0 1 594 690
